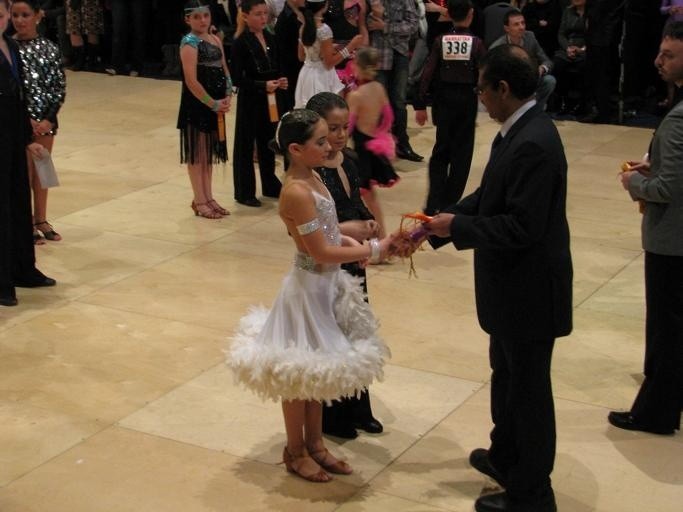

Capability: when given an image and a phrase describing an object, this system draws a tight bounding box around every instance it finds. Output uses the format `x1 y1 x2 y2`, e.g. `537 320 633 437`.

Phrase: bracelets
369 238 381 263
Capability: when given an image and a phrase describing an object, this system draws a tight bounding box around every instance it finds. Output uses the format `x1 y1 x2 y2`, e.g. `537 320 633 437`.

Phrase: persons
177 1 487 235
306 92 382 440
606 17 681 439
473 2 682 125
62 1 186 77
227 108 408 486
1 1 67 305
395 42 574 512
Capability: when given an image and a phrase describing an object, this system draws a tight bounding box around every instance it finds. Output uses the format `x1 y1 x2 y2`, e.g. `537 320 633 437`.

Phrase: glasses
473 81 489 95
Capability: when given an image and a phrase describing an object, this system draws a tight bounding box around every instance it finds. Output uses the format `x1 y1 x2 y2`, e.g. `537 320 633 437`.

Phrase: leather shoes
470 449 505 488
608 411 674 434
476 492 512 511
237 197 261 206
0 297 18 306
327 418 382 439
43 278 57 286
401 151 424 160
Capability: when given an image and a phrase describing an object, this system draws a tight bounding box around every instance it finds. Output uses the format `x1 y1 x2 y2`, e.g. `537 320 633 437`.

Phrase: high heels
283 447 332 482
306 443 354 474
32 221 61 244
191 200 230 218
